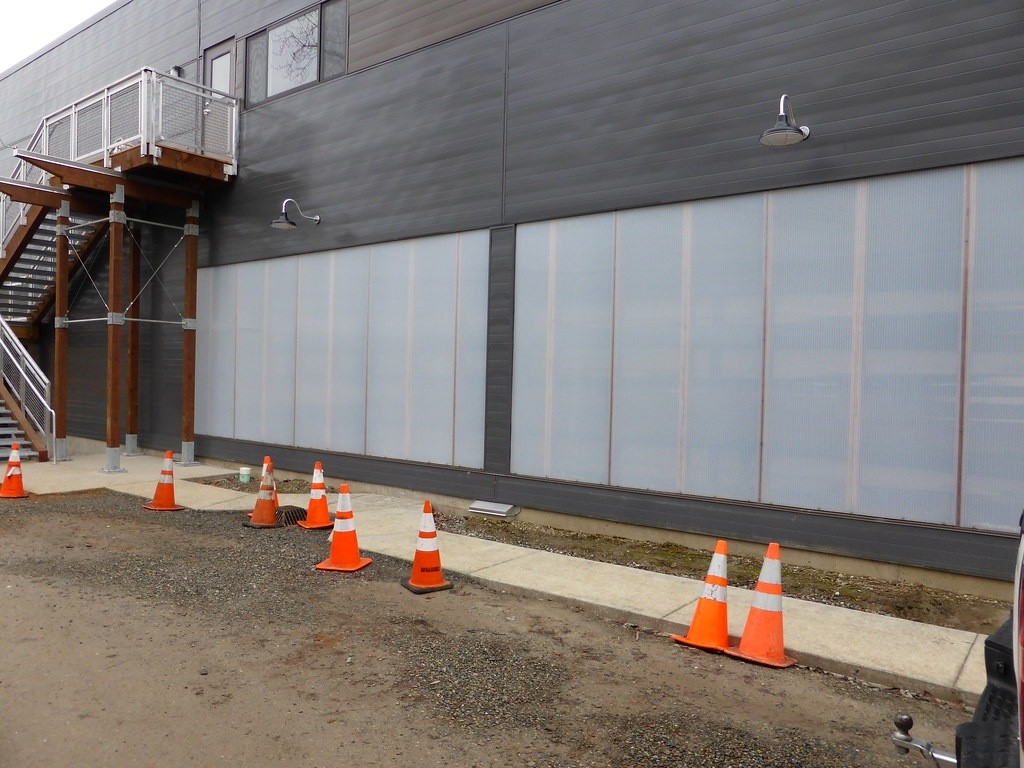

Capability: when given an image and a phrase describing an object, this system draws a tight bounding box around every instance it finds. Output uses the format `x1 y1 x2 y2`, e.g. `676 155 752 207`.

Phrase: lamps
759 94 811 147
171 66 181 78
270 199 321 230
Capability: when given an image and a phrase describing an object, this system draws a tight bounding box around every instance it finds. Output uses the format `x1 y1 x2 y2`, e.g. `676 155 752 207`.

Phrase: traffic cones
242 462 283 530
247 456 279 517
315 483 372 572
400 500 455 595
142 450 185 511
297 461 335 529
670 540 729 653
724 543 797 669
0 443 29 498
